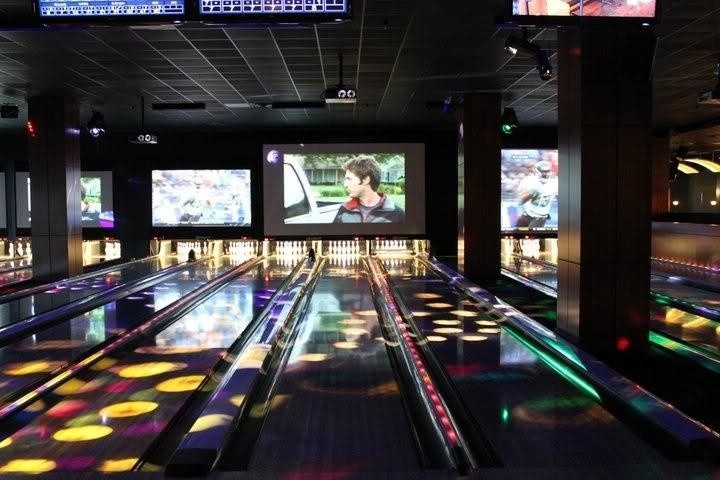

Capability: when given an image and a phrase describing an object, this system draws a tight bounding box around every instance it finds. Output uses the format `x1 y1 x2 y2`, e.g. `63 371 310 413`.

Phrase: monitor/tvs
506 0 664 23
189 0 353 23
31 0 189 24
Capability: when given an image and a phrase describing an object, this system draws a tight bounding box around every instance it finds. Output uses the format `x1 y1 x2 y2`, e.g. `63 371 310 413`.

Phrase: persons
514 159 559 229
332 155 405 224
80 185 101 221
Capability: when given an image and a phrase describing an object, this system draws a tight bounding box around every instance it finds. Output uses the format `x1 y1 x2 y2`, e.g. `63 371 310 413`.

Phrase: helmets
535 160 552 184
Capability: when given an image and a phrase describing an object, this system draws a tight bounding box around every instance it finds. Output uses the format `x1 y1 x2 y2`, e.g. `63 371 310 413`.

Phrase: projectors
697 90 720 105
128 134 159 145
326 88 357 104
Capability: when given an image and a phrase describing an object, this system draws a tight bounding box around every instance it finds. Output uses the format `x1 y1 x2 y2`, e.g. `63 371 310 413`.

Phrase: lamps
499 107 521 136
84 109 107 139
504 27 553 81
